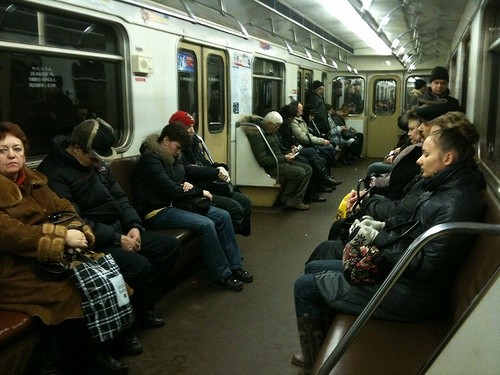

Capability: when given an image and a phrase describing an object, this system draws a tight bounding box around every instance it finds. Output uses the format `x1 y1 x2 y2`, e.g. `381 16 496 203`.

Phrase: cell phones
293 152 299 157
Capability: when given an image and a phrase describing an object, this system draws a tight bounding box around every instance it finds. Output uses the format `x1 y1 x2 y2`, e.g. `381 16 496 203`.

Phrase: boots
292 314 325 375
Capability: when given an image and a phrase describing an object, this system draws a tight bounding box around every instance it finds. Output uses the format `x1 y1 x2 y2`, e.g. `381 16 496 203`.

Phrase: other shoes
280 153 365 210
112 337 144 356
213 276 243 291
92 356 128 375
232 268 253 283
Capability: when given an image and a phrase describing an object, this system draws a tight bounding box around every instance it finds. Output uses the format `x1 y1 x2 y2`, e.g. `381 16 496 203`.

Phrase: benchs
0 154 195 352
313 185 500 375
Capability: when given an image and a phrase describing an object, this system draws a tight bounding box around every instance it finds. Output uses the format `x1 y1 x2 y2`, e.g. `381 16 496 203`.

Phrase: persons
415 66 463 120
276 80 486 265
37 73 78 128
182 55 195 72
167 111 253 264
279 121 489 375
0 122 136 375
239 110 313 211
34 117 166 357
191 82 221 120
131 122 254 292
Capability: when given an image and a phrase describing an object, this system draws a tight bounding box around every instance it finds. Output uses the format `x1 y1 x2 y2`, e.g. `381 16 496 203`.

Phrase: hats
426 111 479 145
414 79 426 89
313 81 324 90
429 66 449 83
168 111 196 127
72 117 116 163
416 104 441 121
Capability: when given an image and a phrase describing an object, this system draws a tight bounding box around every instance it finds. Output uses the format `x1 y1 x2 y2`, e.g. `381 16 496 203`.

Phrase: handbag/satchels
343 220 422 287
173 196 210 216
29 211 76 281
334 190 360 222
207 178 234 194
68 247 136 343
